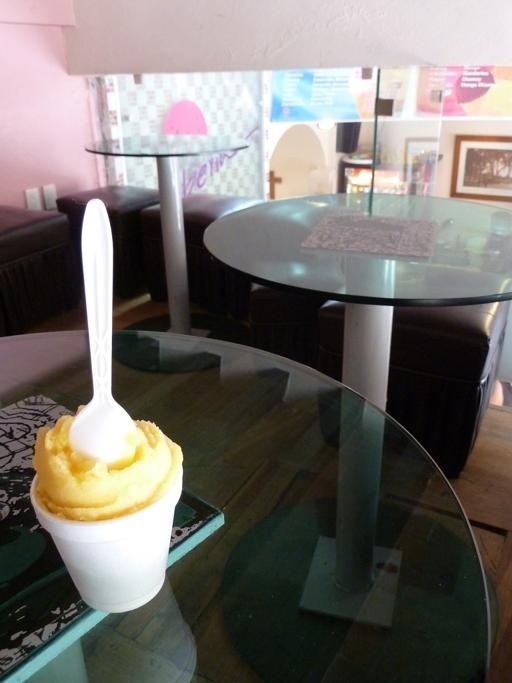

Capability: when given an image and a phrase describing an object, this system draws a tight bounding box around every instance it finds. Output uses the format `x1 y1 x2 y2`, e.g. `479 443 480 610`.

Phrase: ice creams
35 405 183 522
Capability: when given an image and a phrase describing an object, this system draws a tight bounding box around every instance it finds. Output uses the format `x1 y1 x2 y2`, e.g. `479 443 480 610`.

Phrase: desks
0 330 492 682
0 134 510 479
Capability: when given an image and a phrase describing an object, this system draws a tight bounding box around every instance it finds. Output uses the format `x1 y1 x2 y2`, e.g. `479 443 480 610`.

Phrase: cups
28 465 183 616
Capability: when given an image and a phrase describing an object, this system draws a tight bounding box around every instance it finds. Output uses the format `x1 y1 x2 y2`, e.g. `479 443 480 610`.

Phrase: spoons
65 196 144 461
